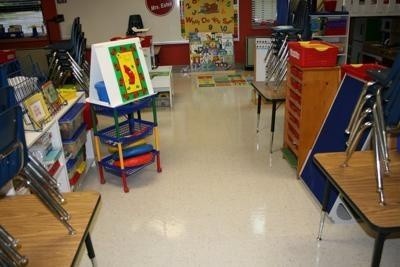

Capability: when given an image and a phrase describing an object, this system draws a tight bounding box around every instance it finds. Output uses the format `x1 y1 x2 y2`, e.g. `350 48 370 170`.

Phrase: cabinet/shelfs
5 92 162 195
282 62 341 179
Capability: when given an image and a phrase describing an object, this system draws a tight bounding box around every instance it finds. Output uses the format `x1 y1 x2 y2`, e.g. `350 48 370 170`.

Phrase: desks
311 148 400 267
247 76 289 154
149 64 174 108
0 191 103 267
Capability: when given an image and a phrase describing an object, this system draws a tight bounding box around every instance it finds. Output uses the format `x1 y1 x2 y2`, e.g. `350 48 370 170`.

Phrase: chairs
264 0 311 91
43 15 91 93
341 36 399 205
